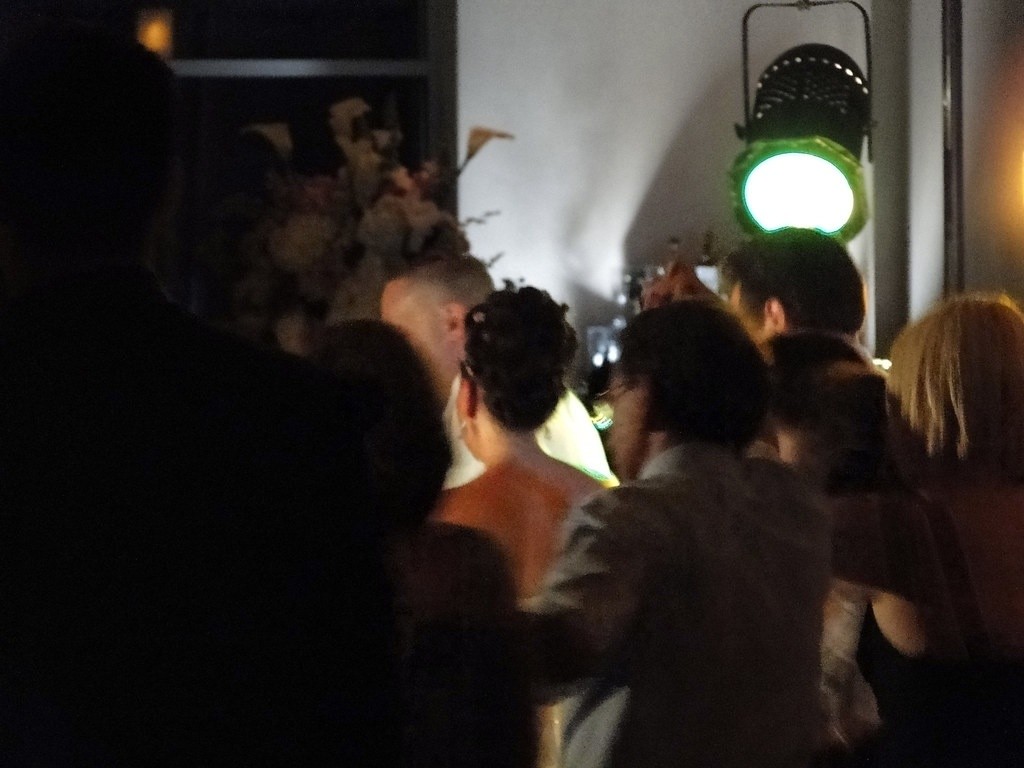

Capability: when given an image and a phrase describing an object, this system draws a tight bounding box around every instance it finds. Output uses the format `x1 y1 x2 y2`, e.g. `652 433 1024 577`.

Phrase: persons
0 25 1024 768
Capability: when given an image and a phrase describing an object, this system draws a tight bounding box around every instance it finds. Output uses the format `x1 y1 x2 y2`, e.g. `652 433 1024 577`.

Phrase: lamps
724 0 876 248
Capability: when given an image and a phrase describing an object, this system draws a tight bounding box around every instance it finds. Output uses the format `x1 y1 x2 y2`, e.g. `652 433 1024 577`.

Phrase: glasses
591 377 641 419
